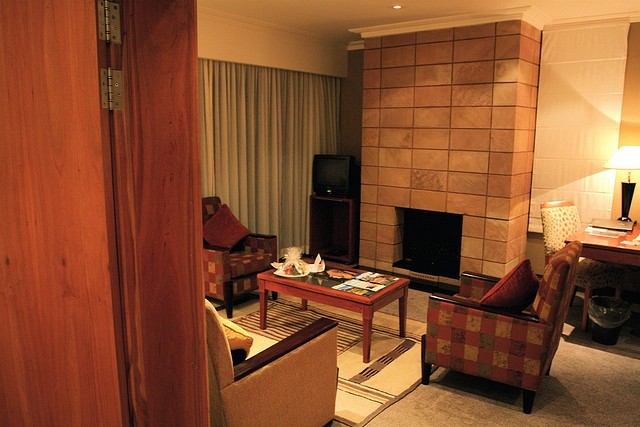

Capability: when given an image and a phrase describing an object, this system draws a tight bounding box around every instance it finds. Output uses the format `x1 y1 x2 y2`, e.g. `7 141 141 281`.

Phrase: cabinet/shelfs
308 195 359 264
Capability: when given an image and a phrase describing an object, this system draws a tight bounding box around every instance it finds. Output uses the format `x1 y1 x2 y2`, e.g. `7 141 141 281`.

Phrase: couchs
421 240 583 398
204 299 339 427
202 196 276 319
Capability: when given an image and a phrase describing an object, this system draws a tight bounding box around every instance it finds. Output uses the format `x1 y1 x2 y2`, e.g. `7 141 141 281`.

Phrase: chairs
541 201 581 266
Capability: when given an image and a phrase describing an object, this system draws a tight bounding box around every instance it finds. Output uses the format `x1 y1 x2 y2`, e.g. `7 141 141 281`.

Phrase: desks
564 217 640 329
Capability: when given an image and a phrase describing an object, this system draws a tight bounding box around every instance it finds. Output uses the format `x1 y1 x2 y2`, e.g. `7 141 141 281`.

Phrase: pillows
232 350 246 366
203 204 251 254
478 258 540 315
220 316 254 367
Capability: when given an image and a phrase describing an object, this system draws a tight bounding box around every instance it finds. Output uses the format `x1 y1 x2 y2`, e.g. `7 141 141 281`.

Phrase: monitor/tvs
312 154 361 196
392 210 461 278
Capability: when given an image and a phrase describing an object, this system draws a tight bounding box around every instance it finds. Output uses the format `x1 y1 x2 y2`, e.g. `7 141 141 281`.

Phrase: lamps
603 146 640 222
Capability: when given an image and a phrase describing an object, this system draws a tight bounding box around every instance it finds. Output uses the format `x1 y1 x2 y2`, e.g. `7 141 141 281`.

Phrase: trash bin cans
586 295 632 345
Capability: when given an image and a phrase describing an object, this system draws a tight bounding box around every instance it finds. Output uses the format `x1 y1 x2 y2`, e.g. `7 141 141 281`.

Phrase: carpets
227 298 440 426
